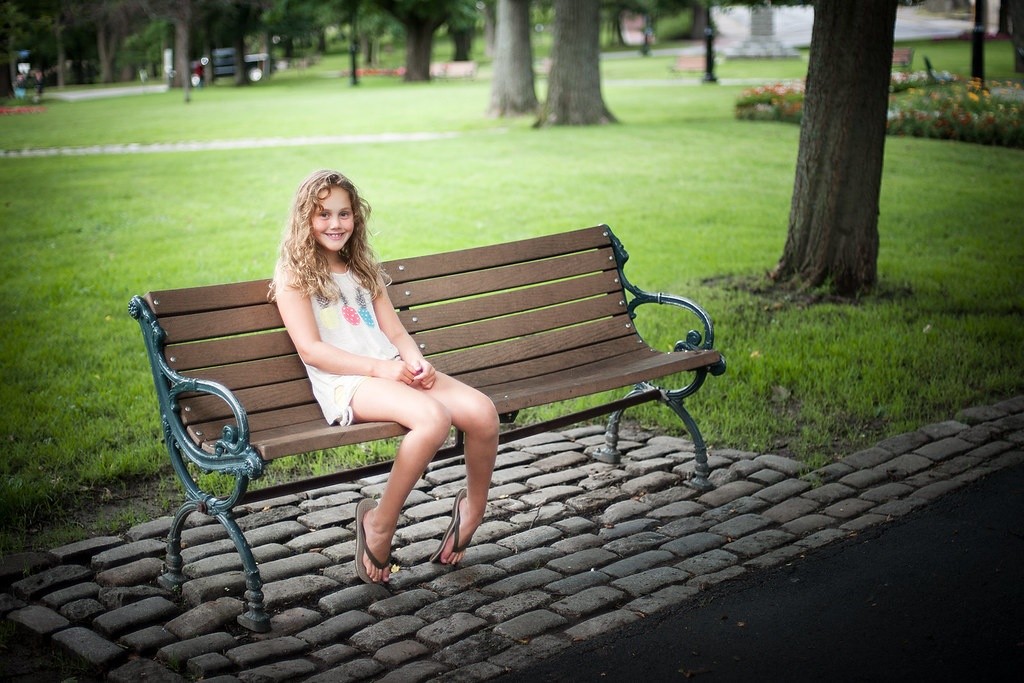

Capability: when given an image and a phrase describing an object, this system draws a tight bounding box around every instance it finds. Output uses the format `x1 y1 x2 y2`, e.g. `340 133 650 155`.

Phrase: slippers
354 498 391 586
430 488 472 564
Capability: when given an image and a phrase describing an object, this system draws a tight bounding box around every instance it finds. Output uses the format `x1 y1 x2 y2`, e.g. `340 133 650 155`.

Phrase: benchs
131 224 724 632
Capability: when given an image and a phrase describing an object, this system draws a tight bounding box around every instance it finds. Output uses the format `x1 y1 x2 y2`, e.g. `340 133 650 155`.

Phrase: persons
31 66 46 104
14 74 28 97
271 168 501 588
